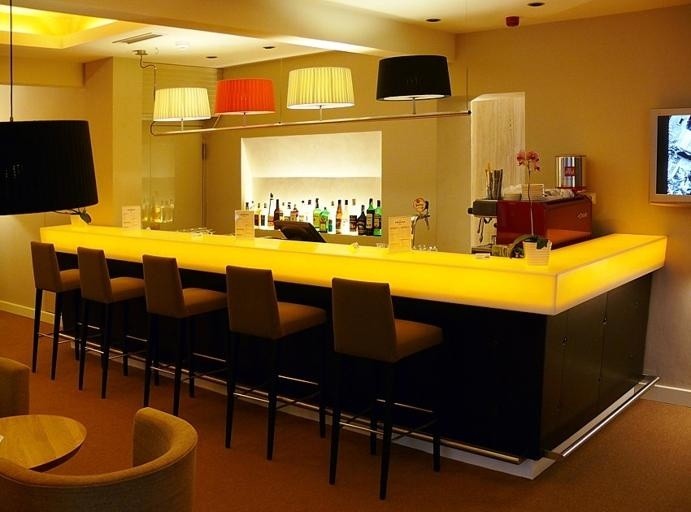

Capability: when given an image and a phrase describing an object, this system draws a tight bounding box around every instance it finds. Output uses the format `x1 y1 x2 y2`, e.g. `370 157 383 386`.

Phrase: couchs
0 404 198 512
0 357 31 419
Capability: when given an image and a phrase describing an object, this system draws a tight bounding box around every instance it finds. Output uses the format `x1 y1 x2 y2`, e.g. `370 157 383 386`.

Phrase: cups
485 168 503 200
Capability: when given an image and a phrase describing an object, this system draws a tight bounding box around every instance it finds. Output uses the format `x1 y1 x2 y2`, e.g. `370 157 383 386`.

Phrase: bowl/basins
504 194 522 201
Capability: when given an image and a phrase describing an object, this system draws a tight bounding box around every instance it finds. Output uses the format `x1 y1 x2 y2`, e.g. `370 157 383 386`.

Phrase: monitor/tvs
274 221 325 243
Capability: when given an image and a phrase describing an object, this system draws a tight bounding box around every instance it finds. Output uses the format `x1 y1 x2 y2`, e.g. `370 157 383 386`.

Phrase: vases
523 241 552 266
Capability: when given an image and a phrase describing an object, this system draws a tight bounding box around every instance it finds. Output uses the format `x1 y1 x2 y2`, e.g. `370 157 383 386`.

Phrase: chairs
76 247 161 400
225 266 329 460
329 277 442 501
31 240 88 381
142 254 227 417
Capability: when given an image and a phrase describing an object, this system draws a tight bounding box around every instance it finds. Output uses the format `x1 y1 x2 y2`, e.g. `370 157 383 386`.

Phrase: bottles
336 200 342 234
327 201 336 234
284 202 292 222
349 199 358 233
306 200 313 224
261 203 269 228
357 205 366 235
274 199 283 230
250 201 255 210
313 198 321 232
268 193 274 227
293 203 299 222
365 199 375 236
254 203 262 228
245 202 249 210
374 200 382 237
299 201 306 222
342 200 349 234
143 191 174 223
290 210 296 221
318 207 330 233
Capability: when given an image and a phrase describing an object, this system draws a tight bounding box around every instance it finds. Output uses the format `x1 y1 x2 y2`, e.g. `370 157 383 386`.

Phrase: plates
521 184 544 200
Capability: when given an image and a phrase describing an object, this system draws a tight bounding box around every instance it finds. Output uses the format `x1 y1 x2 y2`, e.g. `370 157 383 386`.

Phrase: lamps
374 55 451 115
153 87 211 131
215 78 276 126
287 66 355 121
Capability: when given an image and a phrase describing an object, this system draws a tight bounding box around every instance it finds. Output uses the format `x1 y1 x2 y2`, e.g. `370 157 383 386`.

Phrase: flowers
510 149 548 258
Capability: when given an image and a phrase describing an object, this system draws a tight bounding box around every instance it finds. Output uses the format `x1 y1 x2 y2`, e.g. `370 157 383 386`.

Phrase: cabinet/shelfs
249 170 382 239
